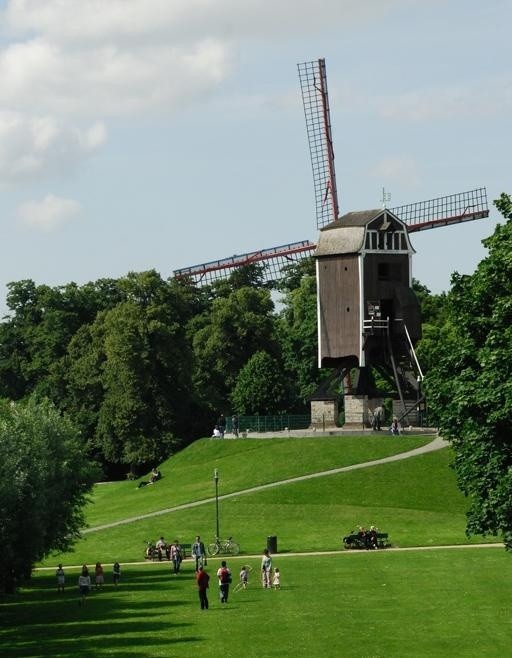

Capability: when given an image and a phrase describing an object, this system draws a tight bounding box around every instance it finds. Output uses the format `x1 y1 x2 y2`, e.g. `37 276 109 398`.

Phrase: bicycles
206 533 240 555
343 523 379 549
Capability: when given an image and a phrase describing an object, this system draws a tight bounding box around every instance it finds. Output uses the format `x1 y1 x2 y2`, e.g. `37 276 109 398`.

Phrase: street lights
212 467 222 540
415 374 426 426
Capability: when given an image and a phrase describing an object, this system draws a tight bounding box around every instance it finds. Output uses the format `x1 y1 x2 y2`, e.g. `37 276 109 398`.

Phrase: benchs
145 543 191 562
349 534 388 550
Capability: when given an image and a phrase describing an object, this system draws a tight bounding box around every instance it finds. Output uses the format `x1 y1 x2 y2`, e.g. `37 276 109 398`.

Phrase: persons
217 561 231 603
390 417 401 436
273 568 281 591
170 540 182 575
156 537 170 561
136 468 161 490
212 413 239 439
353 525 378 550
260 549 272 589
240 566 248 590
192 536 207 573
79 561 121 606
195 566 209 610
56 564 65 593
373 404 385 431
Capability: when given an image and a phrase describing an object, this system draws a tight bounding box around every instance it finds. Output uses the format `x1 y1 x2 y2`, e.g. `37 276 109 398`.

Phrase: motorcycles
143 539 154 561
